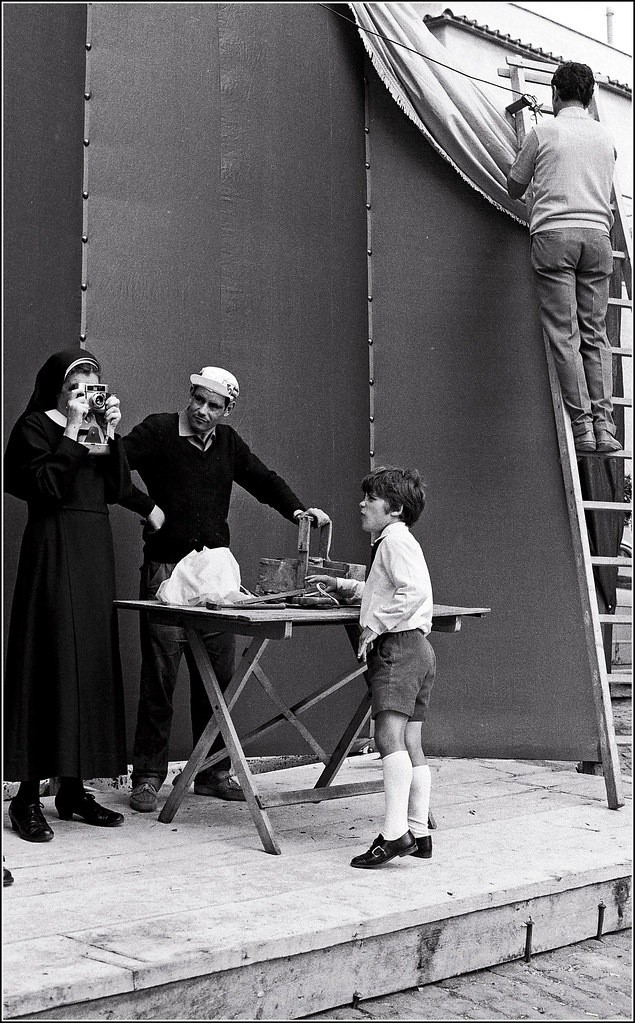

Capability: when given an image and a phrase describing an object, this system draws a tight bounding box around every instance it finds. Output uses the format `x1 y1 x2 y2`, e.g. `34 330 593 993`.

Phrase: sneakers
129 783 156 811
194 769 245 800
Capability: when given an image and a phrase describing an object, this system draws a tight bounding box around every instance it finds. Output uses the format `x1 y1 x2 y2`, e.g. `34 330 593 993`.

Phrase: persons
508 62 622 461
120 366 330 811
3 348 134 841
304 466 438 868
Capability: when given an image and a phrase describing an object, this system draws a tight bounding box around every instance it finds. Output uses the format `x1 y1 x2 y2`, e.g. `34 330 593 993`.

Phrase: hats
190 366 240 402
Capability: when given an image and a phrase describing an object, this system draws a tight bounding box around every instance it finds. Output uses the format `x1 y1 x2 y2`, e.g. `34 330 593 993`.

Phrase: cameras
78 383 111 413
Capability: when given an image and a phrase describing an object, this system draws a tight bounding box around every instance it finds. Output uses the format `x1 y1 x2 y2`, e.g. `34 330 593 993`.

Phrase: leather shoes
351 829 418 868
409 836 432 857
8 796 54 842
55 785 124 827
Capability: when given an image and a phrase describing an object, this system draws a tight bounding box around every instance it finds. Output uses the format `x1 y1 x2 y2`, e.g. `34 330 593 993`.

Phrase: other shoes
2 855 14 885
596 430 622 452
557 430 597 464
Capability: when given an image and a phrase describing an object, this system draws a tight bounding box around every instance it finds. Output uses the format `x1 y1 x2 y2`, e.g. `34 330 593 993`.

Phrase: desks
111 594 492 855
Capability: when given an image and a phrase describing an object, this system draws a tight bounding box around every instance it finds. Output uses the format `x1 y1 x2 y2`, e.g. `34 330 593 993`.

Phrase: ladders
495 61 634 810
